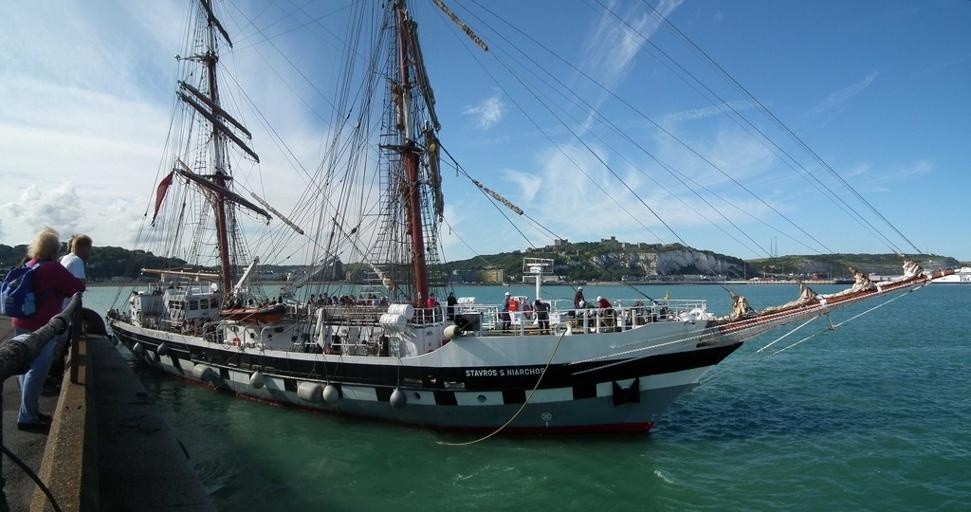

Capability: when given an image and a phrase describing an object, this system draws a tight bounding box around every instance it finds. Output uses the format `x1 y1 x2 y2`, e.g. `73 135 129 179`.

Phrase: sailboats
106 1 958 438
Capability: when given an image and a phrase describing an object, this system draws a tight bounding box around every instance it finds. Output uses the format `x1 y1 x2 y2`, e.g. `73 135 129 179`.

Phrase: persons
447 292 458 320
10 225 87 434
574 287 610 308
307 293 355 306
358 293 388 306
40 233 93 399
502 292 551 334
427 293 438 324
272 297 277 304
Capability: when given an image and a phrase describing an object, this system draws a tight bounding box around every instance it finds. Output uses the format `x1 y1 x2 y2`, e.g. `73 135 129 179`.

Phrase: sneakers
17 412 51 430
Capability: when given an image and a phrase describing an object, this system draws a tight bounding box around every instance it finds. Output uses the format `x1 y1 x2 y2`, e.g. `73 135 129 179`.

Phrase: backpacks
0 258 54 317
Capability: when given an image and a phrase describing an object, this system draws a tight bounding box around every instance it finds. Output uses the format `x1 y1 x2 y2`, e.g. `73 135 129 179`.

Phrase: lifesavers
524 304 532 320
232 338 240 346
